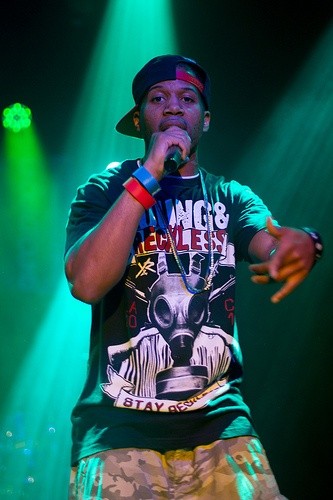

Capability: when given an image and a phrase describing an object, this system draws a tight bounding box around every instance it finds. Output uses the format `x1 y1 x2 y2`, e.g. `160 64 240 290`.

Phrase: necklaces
132 152 220 295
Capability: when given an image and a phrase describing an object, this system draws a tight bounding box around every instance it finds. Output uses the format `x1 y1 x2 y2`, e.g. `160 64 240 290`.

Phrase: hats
114 53 210 139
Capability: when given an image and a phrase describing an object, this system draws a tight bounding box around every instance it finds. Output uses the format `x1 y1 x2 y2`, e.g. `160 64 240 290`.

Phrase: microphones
162 144 181 173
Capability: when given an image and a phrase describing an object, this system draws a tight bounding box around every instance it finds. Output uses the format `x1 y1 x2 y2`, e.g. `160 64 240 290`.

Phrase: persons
65 54 325 500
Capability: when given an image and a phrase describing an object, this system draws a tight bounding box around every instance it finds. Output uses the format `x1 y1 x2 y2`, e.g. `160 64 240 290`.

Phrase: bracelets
131 164 161 200
296 221 324 262
119 174 157 209
